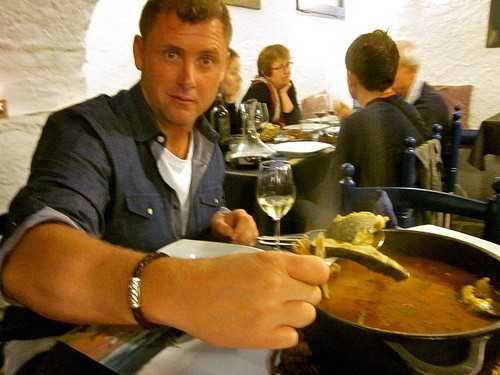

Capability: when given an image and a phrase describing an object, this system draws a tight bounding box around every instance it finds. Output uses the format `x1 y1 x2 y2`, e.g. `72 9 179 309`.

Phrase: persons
195 44 302 236
280 30 452 239
0 0 330 351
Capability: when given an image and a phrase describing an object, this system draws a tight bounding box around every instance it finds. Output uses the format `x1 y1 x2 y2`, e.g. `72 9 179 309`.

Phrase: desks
16 234 500 375
220 115 336 236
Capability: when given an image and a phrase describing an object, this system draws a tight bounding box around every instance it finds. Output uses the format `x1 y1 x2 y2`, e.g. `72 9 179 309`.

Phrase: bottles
210 83 232 152
232 98 274 161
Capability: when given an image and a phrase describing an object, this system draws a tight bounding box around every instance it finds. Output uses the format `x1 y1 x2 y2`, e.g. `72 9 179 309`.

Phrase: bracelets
128 251 170 331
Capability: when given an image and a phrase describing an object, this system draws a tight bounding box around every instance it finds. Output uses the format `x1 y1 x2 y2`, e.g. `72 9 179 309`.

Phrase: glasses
270 62 292 72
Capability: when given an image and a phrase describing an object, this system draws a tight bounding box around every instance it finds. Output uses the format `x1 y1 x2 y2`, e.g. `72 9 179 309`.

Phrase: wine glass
256 160 298 251
312 93 329 136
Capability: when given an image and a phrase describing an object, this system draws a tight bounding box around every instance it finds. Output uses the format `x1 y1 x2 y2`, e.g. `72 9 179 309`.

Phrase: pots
300 228 500 375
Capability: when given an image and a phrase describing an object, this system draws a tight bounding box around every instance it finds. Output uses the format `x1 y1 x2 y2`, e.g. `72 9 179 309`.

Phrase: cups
254 103 269 134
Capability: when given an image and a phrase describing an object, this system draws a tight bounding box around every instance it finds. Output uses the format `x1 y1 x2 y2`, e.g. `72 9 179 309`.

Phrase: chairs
339 103 500 243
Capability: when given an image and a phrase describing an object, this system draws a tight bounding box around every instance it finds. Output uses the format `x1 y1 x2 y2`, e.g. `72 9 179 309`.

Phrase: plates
281 122 332 132
156 238 261 259
270 142 332 158
